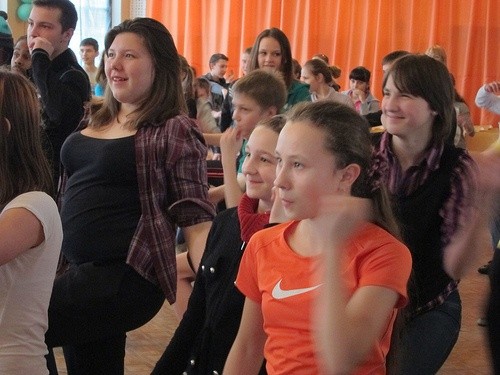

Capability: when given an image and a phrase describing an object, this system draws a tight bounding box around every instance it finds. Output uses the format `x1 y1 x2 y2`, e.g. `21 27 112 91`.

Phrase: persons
0 1 500 375
46 18 214 375
